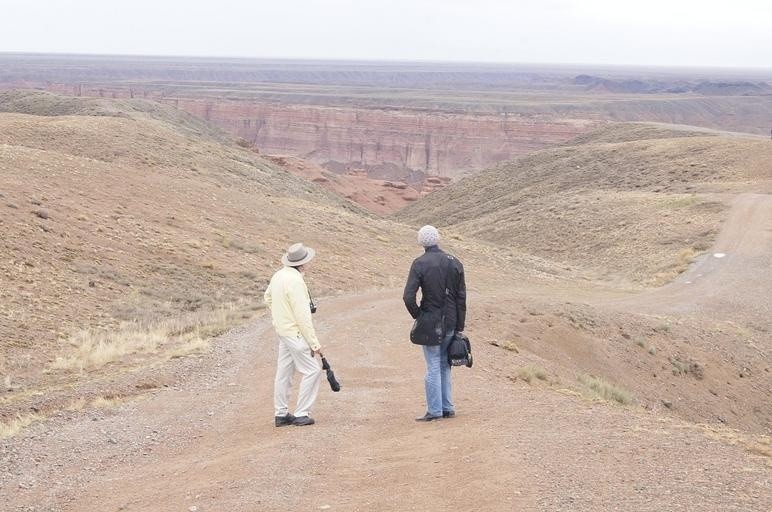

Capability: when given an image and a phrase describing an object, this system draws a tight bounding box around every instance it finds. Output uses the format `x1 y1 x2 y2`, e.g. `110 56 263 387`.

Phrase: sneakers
294 417 313 425
415 413 443 421
441 410 455 418
274 413 295 427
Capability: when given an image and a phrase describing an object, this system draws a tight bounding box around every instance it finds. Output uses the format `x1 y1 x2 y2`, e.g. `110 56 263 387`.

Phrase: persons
265 242 325 427
404 225 467 422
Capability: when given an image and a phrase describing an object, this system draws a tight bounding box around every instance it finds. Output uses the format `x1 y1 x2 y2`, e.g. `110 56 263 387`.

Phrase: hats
419 226 439 247
280 244 315 267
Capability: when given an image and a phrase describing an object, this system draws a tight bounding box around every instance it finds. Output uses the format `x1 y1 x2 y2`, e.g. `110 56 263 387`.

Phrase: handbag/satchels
410 316 444 347
448 334 473 368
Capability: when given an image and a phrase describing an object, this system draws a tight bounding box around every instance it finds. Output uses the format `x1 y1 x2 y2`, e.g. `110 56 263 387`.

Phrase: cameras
310 302 317 313
